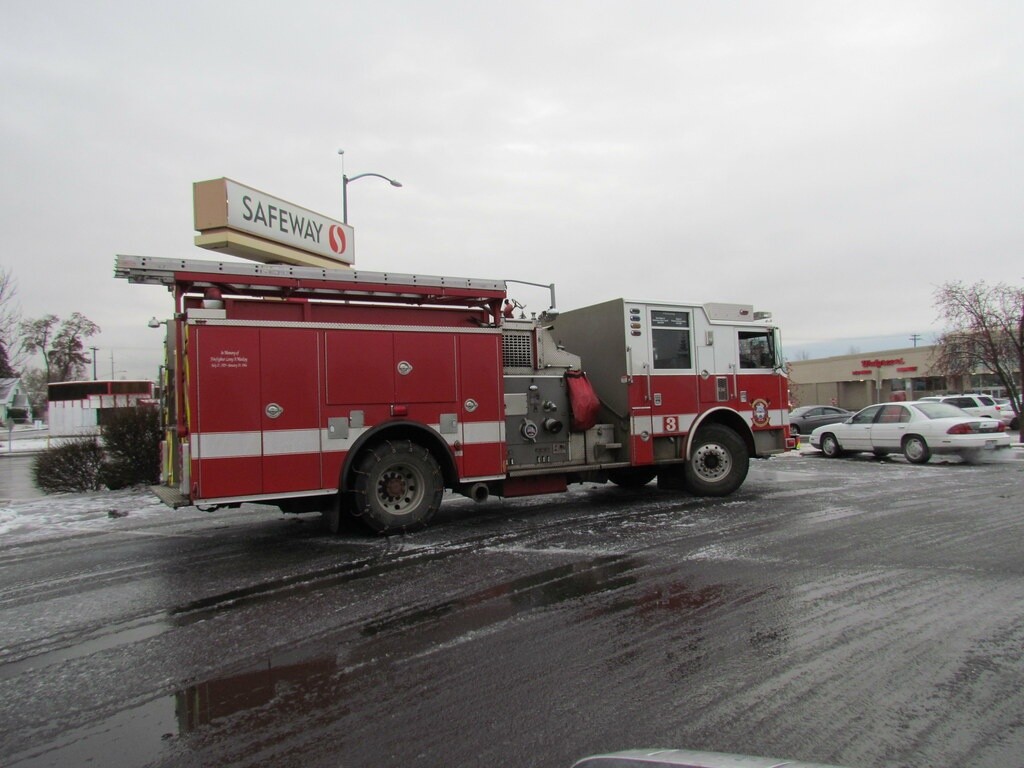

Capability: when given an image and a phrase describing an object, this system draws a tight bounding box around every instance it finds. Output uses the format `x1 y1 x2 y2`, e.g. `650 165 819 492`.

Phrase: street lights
88 347 101 380
337 147 403 226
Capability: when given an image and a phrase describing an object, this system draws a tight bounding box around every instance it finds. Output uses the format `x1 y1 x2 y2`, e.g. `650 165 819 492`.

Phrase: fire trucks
111 251 802 537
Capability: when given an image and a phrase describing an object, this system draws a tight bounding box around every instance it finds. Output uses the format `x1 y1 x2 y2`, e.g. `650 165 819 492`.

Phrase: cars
788 404 856 436
916 393 1024 425
808 400 1012 465
